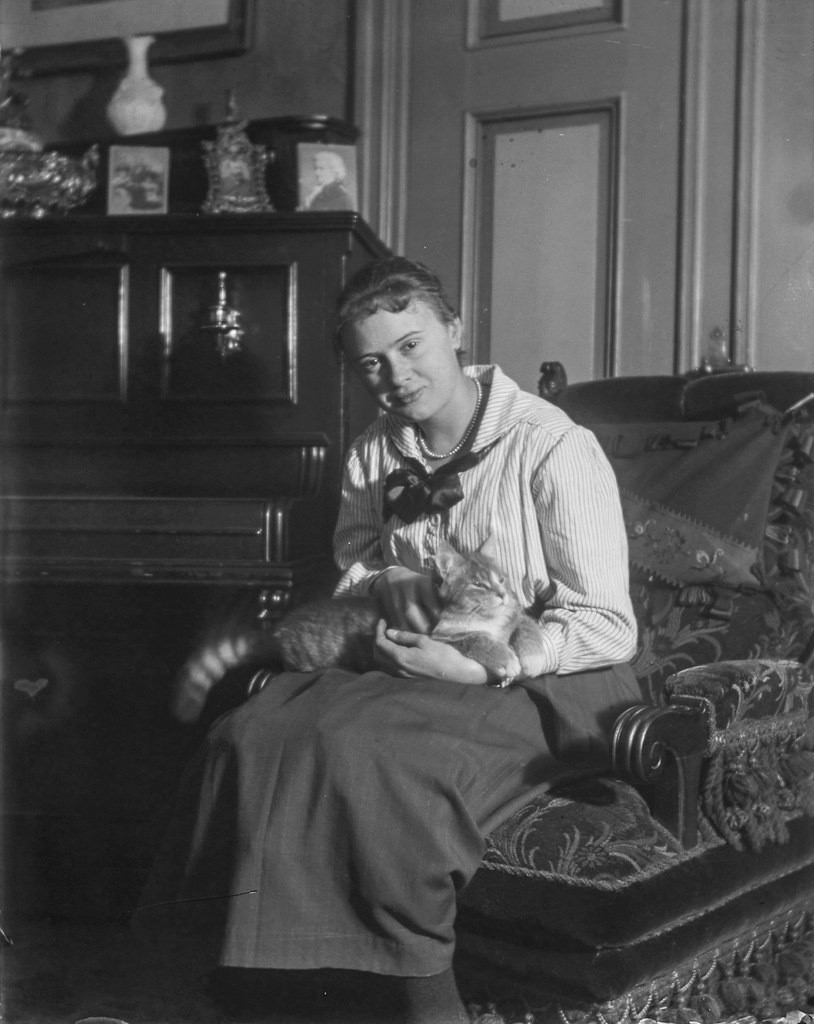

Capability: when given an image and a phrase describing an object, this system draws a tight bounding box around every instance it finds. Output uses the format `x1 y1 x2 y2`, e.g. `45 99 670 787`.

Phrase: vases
105 34 168 137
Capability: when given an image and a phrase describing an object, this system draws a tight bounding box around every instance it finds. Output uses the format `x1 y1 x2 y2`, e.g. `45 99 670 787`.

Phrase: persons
124 259 640 1024
115 161 163 212
305 151 354 213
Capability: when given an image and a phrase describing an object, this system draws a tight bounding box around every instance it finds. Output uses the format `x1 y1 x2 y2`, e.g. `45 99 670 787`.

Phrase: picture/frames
109 144 170 215
0 1 257 78
296 142 359 212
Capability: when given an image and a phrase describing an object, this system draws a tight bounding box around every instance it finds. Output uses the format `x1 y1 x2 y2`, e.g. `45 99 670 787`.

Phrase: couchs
181 370 814 1023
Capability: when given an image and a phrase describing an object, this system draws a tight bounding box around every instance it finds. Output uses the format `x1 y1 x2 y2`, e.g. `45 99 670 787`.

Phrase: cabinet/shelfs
0 209 406 654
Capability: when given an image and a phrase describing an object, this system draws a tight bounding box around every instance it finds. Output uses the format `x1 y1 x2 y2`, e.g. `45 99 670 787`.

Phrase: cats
171 531 545 723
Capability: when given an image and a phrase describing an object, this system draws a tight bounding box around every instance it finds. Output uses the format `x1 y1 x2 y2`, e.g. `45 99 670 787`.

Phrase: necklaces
415 377 484 460
